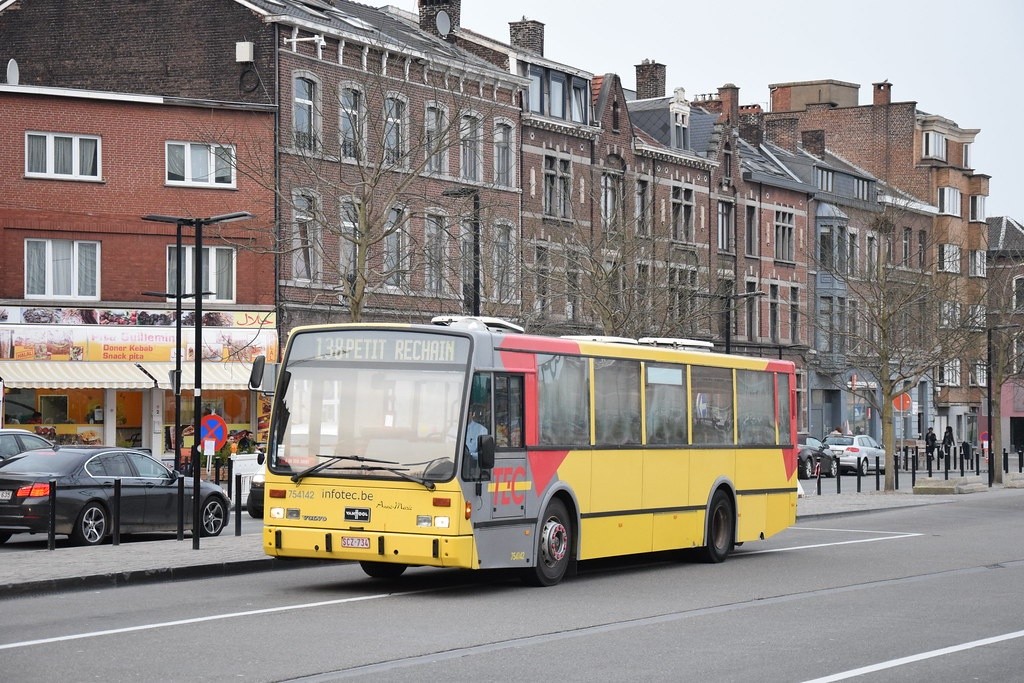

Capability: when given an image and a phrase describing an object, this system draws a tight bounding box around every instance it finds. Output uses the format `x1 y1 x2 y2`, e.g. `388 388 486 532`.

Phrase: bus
246 315 800 587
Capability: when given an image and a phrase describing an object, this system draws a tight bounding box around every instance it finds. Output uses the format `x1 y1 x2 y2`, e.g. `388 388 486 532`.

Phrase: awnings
0 360 262 389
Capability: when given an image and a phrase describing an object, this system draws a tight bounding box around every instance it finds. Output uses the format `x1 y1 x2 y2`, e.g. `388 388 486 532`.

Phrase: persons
226 430 262 454
829 425 843 435
852 425 866 435
941 426 956 453
925 427 936 460
444 404 488 460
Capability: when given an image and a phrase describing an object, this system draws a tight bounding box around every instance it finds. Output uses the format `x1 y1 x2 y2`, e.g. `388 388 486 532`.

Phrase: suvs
820 433 886 475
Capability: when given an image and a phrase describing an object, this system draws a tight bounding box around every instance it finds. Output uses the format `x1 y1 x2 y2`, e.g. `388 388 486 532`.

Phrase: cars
0 445 232 546
797 434 838 480
0 429 57 466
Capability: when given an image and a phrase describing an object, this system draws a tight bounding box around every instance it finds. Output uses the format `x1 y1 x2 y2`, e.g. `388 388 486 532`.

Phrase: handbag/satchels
938 447 944 459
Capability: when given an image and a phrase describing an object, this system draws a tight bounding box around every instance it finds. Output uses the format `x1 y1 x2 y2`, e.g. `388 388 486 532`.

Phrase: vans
247 423 339 519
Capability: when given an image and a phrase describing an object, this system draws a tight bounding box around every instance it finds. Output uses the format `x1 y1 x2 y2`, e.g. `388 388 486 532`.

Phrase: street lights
960 324 1021 488
138 287 218 470
440 186 480 315
137 212 259 549
687 289 766 354
